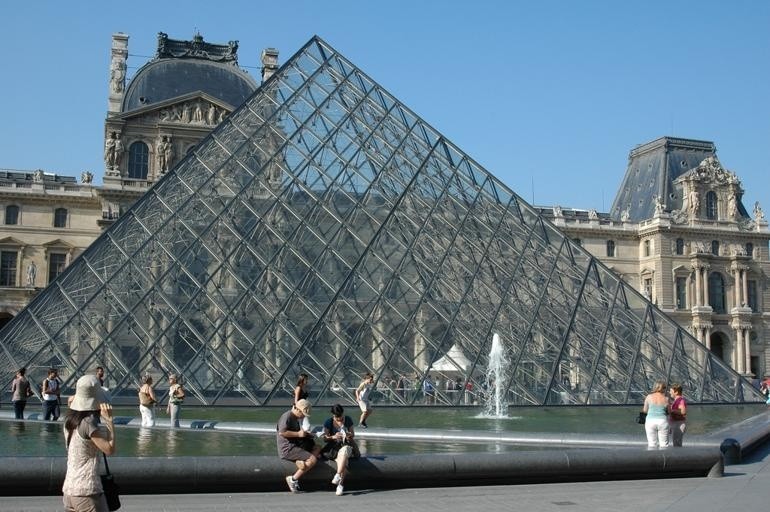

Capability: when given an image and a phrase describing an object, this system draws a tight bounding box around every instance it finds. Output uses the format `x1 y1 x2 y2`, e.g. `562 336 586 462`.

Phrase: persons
11 368 33 419
62 374 116 512
166 373 184 428
322 404 360 496
759 372 770 405
293 374 308 409
138 375 158 427
355 373 375 428
277 399 322 493
96 366 105 424
643 380 671 450
384 373 474 406
42 370 62 421
669 383 687 447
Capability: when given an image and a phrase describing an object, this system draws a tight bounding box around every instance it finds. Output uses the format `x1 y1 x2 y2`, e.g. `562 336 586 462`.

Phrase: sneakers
332 473 341 484
296 460 305 470
358 423 368 428
336 484 343 496
286 476 299 494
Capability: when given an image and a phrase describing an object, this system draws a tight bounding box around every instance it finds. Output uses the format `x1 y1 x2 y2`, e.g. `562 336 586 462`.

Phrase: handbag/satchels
138 391 154 406
170 389 184 404
99 474 120 511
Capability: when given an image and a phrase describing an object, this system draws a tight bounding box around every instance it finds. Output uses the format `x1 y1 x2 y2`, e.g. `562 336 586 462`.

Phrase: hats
70 374 112 412
295 398 313 417
